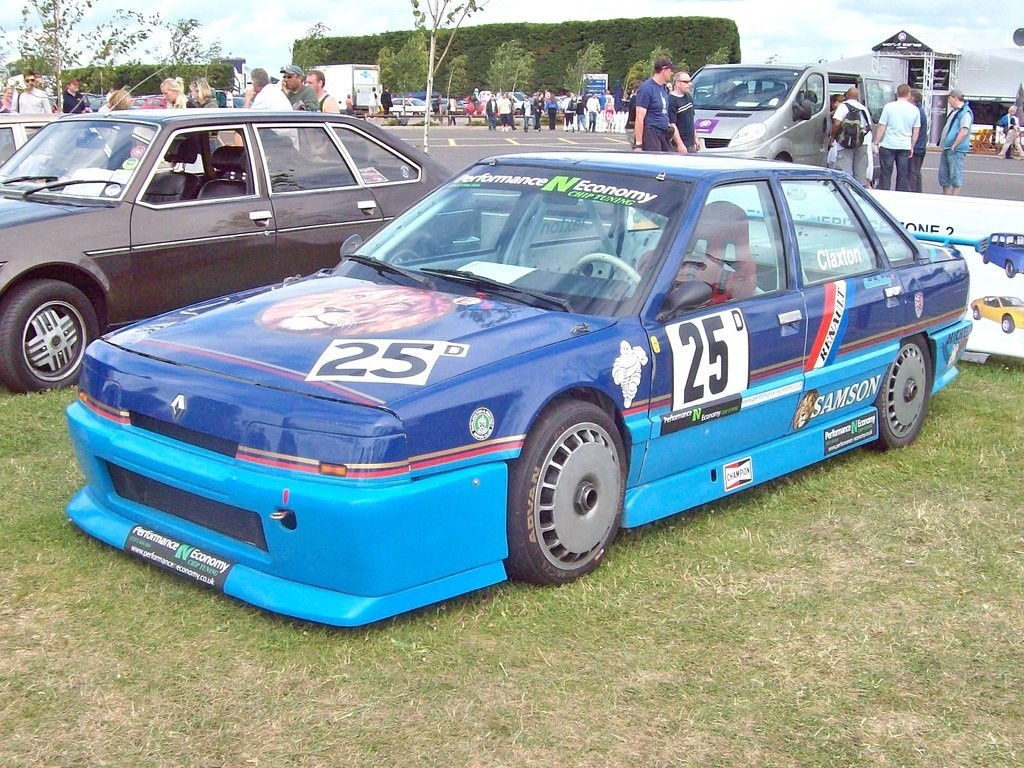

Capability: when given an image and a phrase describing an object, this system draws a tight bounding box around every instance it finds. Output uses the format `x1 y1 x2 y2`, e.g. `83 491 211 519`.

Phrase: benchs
261 133 403 191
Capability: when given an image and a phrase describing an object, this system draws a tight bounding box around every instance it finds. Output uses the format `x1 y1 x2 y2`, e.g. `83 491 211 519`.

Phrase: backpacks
835 103 871 149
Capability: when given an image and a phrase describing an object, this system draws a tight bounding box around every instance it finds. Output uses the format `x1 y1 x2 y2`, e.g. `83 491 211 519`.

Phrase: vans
479 91 532 115
687 63 896 189
983 233 1024 278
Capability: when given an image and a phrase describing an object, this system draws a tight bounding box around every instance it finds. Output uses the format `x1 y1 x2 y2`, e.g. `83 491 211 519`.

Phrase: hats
946 89 964 100
654 58 676 71
280 65 305 76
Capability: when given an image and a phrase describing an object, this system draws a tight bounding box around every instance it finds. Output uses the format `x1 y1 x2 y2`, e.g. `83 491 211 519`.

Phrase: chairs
633 201 756 305
198 145 246 198
738 83 786 104
142 140 204 204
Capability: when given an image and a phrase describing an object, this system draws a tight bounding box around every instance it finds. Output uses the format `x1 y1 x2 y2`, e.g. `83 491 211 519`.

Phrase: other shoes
487 125 613 133
1019 156 1024 160
1006 155 1014 159
997 153 1005 157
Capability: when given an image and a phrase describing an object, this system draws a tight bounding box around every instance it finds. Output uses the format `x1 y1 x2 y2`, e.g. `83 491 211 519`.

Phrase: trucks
304 64 380 110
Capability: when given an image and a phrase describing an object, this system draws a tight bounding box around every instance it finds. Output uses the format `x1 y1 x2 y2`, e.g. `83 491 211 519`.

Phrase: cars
0 114 480 394
388 98 463 118
971 296 1024 333
62 152 972 627
81 92 168 109
233 97 253 108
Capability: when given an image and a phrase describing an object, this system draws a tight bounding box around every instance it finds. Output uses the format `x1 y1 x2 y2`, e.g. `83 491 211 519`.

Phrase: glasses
25 79 35 82
283 75 298 79
678 80 691 84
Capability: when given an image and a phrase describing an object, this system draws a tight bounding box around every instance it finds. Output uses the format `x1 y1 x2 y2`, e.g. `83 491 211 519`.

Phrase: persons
160 77 233 175
368 86 379 116
486 90 615 132
244 66 341 154
0 86 14 113
433 93 443 124
997 105 1023 160
625 57 701 154
346 94 353 115
56 78 86 113
98 81 133 113
18 70 53 114
380 84 393 116
449 96 457 126
823 85 927 192
936 89 973 194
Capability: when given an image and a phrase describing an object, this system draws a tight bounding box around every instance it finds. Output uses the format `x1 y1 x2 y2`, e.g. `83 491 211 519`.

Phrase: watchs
873 141 879 145
635 142 642 145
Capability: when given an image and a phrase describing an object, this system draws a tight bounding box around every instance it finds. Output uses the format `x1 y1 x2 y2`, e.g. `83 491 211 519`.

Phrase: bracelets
634 146 643 148
